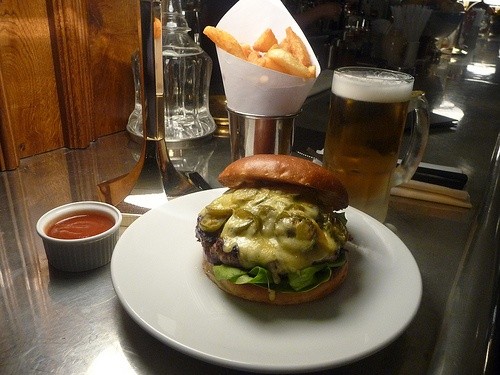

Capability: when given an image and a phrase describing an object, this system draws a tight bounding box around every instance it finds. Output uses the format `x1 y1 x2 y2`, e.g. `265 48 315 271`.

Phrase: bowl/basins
422 10 466 40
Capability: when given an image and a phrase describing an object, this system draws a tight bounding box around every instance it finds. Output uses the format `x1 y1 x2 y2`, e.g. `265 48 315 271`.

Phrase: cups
470 25 479 49
322 66 430 223
402 41 420 67
224 103 304 162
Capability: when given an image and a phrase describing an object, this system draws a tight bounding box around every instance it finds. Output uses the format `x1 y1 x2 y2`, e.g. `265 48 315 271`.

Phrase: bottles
125 0 218 143
384 29 405 65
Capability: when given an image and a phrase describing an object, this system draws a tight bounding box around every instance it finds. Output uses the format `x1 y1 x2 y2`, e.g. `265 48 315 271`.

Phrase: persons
284 0 342 39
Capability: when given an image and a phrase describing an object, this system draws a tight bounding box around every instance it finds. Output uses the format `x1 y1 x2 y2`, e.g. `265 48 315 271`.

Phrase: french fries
203 26 317 80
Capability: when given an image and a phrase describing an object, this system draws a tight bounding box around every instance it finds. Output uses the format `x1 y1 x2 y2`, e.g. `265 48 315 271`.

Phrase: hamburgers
195 154 353 304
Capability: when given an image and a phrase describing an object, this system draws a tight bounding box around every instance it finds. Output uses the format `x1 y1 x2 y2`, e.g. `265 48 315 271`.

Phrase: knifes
293 148 468 190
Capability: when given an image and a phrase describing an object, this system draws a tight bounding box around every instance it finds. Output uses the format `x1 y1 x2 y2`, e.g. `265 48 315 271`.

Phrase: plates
209 95 229 131
110 187 423 374
35 201 123 272
